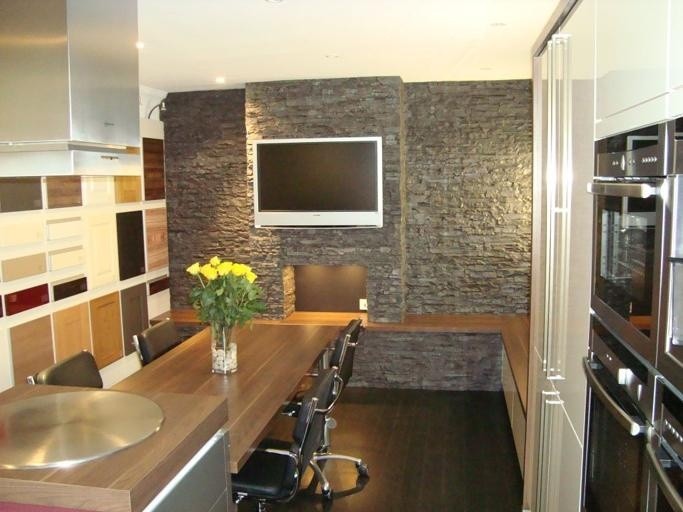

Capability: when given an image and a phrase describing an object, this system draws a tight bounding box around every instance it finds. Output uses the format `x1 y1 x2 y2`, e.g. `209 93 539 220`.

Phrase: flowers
185 255 269 349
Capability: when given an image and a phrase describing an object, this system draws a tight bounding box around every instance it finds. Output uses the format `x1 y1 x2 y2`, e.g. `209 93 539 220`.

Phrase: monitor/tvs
253 136 383 229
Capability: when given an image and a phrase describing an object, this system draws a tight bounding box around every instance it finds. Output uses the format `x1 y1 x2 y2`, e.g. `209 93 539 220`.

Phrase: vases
209 320 239 375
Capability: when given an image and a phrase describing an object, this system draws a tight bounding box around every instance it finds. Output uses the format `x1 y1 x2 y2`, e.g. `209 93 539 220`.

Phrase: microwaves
588 121 668 366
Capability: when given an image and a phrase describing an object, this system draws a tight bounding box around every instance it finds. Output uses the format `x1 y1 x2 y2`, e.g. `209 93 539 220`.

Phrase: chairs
230 318 370 511
27 316 181 390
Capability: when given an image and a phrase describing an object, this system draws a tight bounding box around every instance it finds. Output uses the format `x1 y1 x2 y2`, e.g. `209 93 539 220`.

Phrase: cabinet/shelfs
524 0 594 511
594 2 671 121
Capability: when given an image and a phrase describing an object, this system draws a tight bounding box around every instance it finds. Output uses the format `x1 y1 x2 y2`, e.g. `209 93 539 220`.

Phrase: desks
107 324 339 474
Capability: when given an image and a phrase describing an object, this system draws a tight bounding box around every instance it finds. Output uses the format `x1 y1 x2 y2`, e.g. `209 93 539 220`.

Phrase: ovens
579 313 683 512
